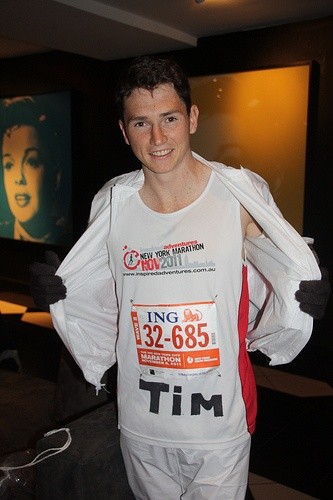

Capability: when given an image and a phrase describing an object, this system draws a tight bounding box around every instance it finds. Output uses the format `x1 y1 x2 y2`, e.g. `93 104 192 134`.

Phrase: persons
0 96 67 245
23 54 332 499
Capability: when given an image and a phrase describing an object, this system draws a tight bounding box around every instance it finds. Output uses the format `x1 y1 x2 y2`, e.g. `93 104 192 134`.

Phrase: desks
250 364 333 398
